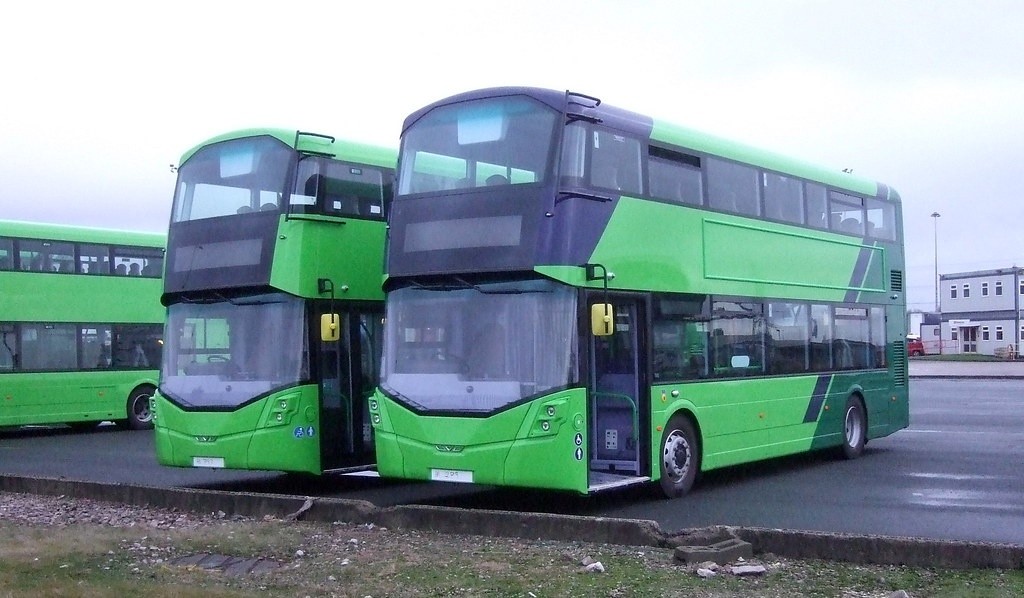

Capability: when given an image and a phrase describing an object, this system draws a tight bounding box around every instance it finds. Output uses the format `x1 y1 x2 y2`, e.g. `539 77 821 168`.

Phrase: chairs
455 171 875 236
0 253 157 279
236 193 372 216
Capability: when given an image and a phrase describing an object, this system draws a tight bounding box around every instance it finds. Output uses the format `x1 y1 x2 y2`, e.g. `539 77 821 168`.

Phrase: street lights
930 211 941 312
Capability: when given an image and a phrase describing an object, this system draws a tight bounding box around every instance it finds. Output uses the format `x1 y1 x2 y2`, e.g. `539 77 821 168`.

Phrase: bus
0 219 229 437
367 85 909 501
148 126 537 482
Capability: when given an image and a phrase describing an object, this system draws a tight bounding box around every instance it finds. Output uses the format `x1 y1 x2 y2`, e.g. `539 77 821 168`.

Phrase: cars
907 337 925 356
